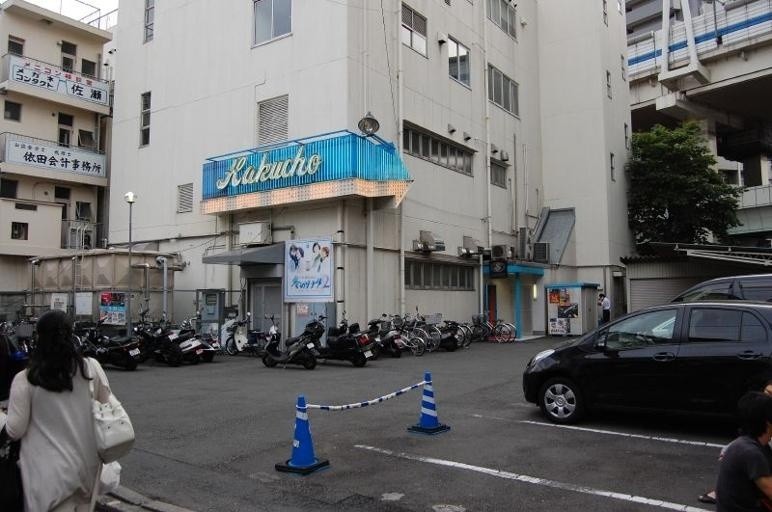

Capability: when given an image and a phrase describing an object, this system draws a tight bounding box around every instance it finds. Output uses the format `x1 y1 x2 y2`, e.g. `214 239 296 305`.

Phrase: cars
522 299 771 424
639 311 696 338
0 335 28 400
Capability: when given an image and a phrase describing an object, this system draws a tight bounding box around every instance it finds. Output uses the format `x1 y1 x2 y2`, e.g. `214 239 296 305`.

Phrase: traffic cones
406 371 450 435
275 394 330 475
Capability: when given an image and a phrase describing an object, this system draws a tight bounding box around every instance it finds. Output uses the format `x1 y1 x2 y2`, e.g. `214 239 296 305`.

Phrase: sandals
698 493 717 505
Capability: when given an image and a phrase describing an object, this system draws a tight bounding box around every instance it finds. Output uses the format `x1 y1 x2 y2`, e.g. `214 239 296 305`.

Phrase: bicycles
388 303 518 355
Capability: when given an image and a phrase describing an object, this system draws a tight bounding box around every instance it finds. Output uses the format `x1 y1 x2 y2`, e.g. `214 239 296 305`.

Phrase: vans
664 273 772 304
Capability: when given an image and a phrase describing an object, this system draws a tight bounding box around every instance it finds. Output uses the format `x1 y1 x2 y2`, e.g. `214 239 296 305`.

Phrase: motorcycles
259 314 322 371
367 313 401 358
302 314 374 368
0 309 220 367
337 308 379 363
225 312 269 356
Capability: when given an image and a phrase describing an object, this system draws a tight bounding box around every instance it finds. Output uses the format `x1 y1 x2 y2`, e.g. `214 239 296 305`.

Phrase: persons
294 247 309 270
697 384 771 503
598 294 611 324
287 245 298 271
307 243 320 269
317 247 330 271
716 391 771 511
7 311 112 512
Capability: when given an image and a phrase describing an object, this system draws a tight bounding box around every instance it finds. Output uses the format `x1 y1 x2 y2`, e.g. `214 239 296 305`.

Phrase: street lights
124 191 138 338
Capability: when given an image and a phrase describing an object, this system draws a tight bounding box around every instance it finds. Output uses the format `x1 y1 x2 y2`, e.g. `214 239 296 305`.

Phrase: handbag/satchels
82 356 136 465
0 428 24 510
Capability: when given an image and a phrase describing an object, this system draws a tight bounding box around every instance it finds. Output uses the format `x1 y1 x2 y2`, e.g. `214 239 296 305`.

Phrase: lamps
358 111 379 138
